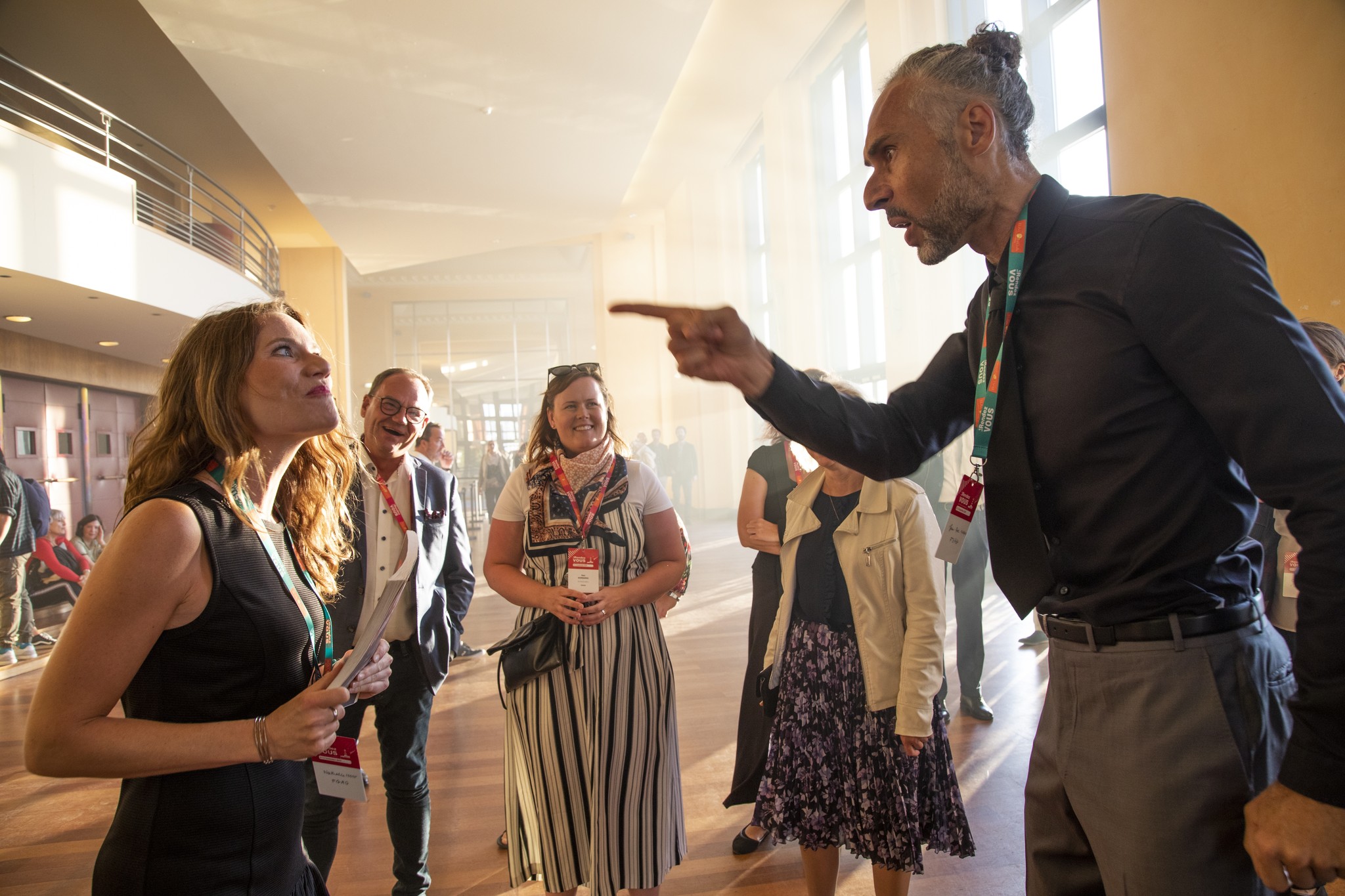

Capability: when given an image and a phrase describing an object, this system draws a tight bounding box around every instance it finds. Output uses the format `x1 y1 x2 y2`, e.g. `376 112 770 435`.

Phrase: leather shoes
960 692 994 721
939 699 951 724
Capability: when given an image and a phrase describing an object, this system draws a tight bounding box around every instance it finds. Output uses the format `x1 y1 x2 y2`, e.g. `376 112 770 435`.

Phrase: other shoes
1018 631 1049 645
732 822 771 856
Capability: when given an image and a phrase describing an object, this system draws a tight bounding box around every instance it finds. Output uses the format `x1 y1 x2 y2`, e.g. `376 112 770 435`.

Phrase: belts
937 502 984 512
1037 587 1266 647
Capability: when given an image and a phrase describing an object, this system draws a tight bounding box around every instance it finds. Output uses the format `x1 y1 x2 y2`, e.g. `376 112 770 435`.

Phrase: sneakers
452 640 486 661
30 632 58 646
0 647 18 666
13 641 38 659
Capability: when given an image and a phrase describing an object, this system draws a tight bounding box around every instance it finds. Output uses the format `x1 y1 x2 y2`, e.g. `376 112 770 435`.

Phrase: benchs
33 600 74 630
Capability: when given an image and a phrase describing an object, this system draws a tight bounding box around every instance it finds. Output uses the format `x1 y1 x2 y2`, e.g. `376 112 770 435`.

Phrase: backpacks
16 474 51 539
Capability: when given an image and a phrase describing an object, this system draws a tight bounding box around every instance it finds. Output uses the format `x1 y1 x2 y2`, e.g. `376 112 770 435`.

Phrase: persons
722 368 828 855
904 423 995 723
479 424 701 539
1247 321 1345 671
495 505 692 851
69 514 106 571
0 447 36 665
482 362 686 896
415 422 487 660
302 368 476 896
751 381 976 894
25 299 395 896
611 20 1345 896
24 509 91 608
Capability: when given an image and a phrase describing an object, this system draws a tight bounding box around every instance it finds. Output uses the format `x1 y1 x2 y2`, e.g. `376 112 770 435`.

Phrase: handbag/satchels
755 663 781 719
486 610 565 710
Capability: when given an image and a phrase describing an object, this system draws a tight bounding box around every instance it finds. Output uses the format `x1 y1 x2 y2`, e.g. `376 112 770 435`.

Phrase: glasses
371 394 427 424
547 362 602 390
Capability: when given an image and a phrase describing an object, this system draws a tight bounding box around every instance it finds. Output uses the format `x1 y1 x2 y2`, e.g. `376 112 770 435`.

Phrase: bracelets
83 569 90 573
441 467 451 471
254 715 274 765
667 591 680 602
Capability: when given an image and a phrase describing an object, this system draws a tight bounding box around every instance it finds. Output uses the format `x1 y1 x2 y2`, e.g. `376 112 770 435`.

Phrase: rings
329 706 338 722
600 610 605 616
